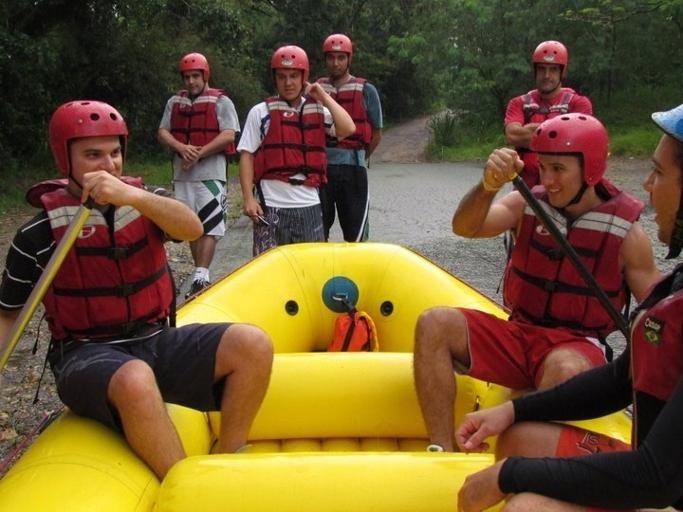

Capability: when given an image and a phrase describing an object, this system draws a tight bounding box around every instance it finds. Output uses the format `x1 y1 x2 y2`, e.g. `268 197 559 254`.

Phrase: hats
45 98 129 178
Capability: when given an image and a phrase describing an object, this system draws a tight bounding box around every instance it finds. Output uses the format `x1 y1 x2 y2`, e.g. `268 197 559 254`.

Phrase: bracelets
481 175 500 192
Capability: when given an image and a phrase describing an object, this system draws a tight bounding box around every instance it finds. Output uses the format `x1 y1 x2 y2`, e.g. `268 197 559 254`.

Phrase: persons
309 34 384 243
0 99 274 481
413 112 664 452
236 44 357 257
155 53 242 296
504 39 593 262
457 104 683 511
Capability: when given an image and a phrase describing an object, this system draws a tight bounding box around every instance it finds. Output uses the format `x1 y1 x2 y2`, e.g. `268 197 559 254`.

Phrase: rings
184 165 186 167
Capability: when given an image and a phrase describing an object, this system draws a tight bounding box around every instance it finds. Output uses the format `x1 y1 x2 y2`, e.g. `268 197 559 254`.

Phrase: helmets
321 33 353 65
178 52 210 83
528 111 610 186
532 38 569 81
650 103 683 143
270 44 311 86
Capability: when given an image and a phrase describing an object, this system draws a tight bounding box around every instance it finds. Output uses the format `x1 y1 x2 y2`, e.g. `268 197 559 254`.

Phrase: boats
0 233 683 511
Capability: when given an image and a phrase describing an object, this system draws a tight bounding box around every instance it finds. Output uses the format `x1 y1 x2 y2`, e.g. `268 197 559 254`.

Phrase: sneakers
184 279 211 298
